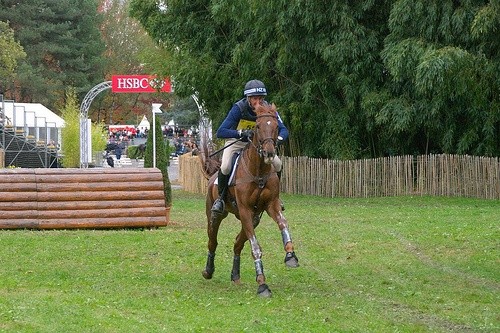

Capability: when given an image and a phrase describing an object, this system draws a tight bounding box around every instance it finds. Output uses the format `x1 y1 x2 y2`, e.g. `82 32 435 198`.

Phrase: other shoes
211 201 223 211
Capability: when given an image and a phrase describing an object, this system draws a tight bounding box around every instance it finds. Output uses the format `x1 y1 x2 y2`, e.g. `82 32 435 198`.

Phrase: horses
196 99 300 299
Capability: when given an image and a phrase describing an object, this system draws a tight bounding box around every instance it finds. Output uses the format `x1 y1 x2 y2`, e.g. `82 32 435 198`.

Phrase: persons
105 125 199 168
210 80 288 213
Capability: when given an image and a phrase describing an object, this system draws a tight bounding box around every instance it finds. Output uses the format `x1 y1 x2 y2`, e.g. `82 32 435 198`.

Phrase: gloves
276 135 284 146
236 129 253 141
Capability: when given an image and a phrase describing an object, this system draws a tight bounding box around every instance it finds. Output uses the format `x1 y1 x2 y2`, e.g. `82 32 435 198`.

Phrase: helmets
244 80 267 96
